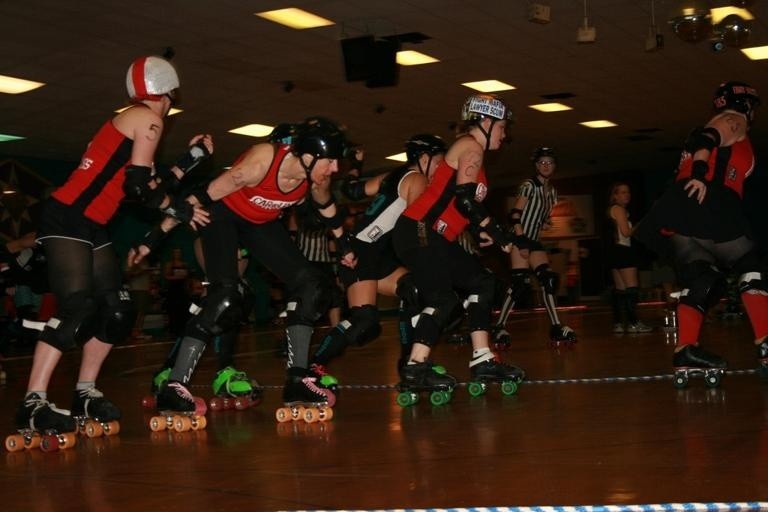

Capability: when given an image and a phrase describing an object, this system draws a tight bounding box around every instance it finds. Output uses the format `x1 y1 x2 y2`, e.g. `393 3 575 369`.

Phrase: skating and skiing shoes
488 324 515 354
4 391 79 451
673 343 729 388
396 356 457 406
276 355 338 422
446 324 471 344
715 308 749 322
208 365 262 411
70 389 122 437
754 336 768 384
467 350 527 398
141 366 208 432
548 323 578 347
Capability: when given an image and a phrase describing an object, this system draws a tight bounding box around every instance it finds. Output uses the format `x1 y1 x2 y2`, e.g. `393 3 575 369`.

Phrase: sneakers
627 320 654 334
611 320 625 334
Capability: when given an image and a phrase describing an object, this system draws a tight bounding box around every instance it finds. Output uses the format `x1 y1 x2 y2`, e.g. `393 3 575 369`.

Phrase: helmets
292 114 345 161
124 55 180 102
406 133 446 164
266 122 296 146
712 79 761 114
531 146 562 164
460 92 516 125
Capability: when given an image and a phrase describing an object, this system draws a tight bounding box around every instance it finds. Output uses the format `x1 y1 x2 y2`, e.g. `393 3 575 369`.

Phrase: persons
147 162 260 399
11 54 220 441
2 142 766 361
125 112 350 419
654 79 768 370
392 91 530 386
303 128 448 390
